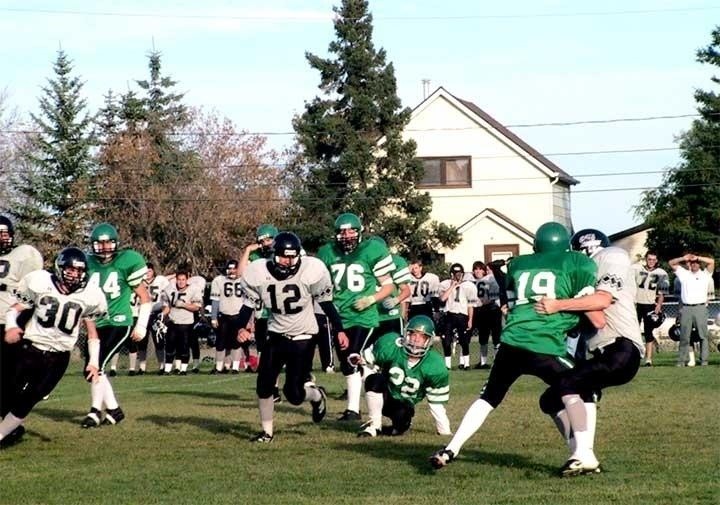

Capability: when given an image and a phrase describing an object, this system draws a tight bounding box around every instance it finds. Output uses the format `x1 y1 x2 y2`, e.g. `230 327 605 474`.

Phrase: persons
336 235 412 402
316 212 398 423
0 247 109 448
0 214 44 345
77 221 154 428
347 314 455 438
531 227 649 477
235 231 350 444
429 220 608 471
236 221 335 402
76 252 715 380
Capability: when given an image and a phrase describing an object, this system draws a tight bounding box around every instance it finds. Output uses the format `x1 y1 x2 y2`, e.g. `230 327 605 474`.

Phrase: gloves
352 295 376 311
383 297 399 309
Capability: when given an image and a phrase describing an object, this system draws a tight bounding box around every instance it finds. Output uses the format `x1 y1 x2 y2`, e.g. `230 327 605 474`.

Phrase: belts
682 303 705 307
286 334 312 340
32 346 56 356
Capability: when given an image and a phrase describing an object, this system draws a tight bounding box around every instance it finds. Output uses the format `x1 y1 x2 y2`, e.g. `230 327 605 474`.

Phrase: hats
335 213 361 252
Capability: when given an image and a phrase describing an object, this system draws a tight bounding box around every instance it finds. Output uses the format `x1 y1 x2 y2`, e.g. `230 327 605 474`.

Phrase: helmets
401 314 435 358
450 264 464 282
273 232 301 274
669 325 680 341
257 224 279 252
54 248 89 291
532 222 569 252
91 223 118 259
226 260 238 279
0 215 14 254
647 310 666 328
571 229 611 256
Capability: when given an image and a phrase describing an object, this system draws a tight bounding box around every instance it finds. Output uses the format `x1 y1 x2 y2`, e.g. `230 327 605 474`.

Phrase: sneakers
701 361 708 365
382 426 397 436
102 408 124 425
109 366 256 376
249 431 275 444
558 454 602 477
675 362 686 367
335 408 361 421
81 412 101 428
310 386 326 423
1 425 24 447
475 362 490 368
688 361 696 366
464 365 471 369
430 449 454 468
357 426 382 437
458 365 464 368
645 360 652 366
274 395 282 403
